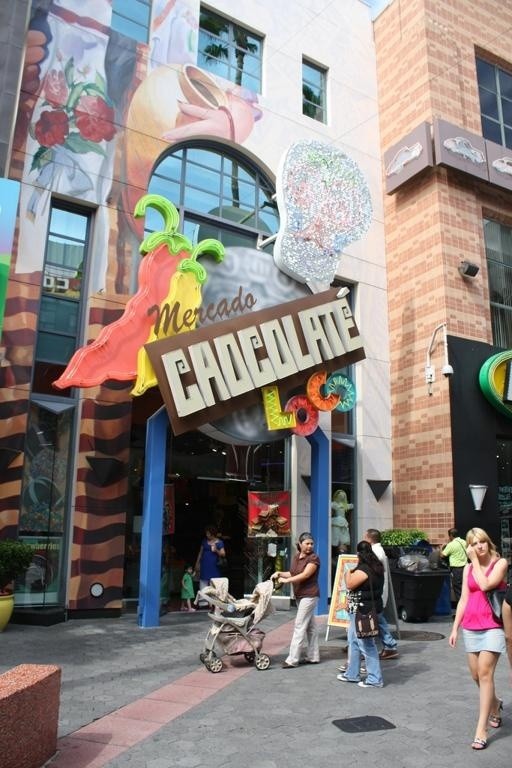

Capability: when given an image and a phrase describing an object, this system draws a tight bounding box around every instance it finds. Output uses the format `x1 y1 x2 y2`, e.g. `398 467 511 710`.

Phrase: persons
336 541 384 687
195 524 227 610
499 581 511 674
338 661 367 674
179 562 197 614
447 527 508 750
269 532 322 668
330 489 353 548
440 529 468 621
341 529 399 661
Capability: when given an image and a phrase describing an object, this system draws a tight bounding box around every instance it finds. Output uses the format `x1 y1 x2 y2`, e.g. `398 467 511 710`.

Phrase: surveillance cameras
441 364 453 377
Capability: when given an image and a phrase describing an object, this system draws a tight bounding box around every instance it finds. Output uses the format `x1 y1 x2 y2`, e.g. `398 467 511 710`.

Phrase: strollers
190 574 287 674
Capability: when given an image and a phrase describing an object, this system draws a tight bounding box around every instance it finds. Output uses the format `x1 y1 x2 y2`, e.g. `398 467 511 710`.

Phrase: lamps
424 319 455 395
469 483 488 512
458 259 480 277
366 479 392 503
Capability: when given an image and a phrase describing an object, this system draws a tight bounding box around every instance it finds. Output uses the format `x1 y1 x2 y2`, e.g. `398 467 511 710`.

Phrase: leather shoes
283 660 296 668
303 658 320 664
379 647 399 658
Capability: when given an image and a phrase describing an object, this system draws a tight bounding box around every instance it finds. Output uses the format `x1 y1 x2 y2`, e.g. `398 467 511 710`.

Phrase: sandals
180 607 187 610
188 608 196 612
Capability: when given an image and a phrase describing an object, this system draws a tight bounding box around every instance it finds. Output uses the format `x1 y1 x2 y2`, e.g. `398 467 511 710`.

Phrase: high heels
472 723 489 750
488 697 504 728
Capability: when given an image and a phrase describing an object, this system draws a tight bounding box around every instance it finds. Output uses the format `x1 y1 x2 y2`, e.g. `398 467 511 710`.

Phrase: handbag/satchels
353 602 381 638
484 557 511 624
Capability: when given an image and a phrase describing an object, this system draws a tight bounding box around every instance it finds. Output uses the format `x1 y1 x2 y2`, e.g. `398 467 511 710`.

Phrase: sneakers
336 663 362 682
358 678 382 688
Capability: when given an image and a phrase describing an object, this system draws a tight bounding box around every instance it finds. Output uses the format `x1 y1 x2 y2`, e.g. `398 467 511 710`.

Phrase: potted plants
0 539 32 633
